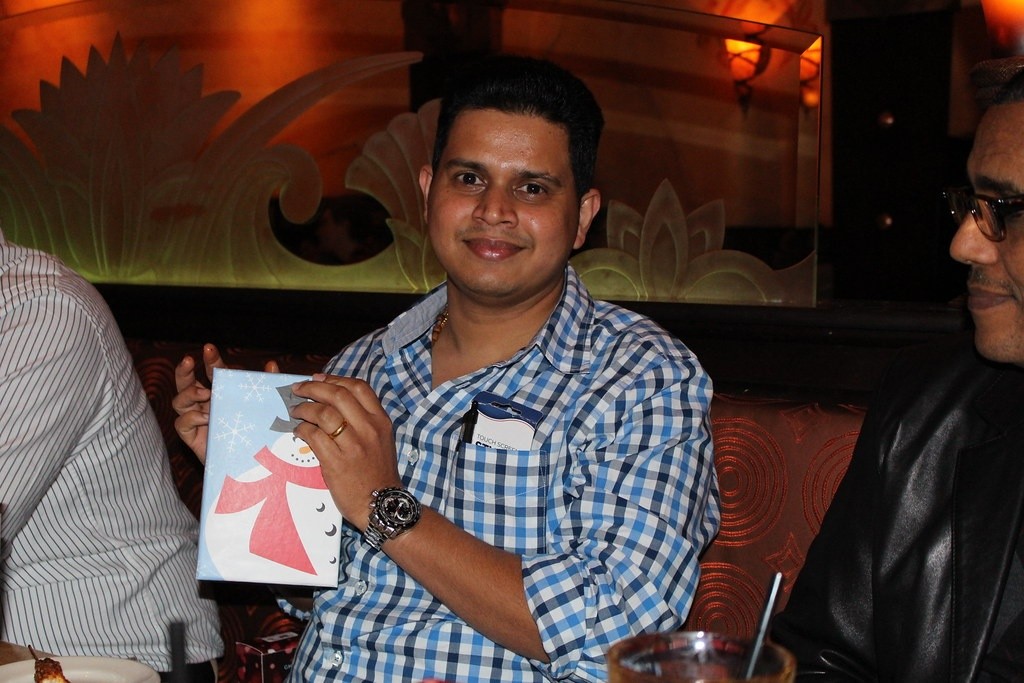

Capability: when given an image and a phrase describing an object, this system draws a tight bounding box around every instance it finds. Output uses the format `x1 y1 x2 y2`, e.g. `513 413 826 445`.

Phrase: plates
0 657 161 683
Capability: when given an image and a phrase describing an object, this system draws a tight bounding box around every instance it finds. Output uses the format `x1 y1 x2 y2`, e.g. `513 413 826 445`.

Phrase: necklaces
429 303 449 348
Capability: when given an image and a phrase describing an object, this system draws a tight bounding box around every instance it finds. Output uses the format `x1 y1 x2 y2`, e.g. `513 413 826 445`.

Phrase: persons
174 53 720 682
767 67 1024 683
0 227 226 682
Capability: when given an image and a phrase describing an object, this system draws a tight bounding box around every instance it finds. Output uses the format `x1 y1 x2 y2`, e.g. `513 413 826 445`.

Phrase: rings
328 420 347 439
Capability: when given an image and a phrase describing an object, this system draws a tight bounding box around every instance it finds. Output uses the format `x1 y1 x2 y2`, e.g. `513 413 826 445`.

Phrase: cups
606 631 794 683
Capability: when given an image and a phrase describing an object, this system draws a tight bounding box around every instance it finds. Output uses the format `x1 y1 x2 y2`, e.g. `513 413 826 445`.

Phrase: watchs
363 486 422 552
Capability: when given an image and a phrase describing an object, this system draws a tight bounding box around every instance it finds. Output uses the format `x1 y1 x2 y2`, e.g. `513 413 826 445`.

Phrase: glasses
943 188 1024 243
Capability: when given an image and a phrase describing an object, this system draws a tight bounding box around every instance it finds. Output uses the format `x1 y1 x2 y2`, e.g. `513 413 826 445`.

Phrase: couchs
135 341 867 683
